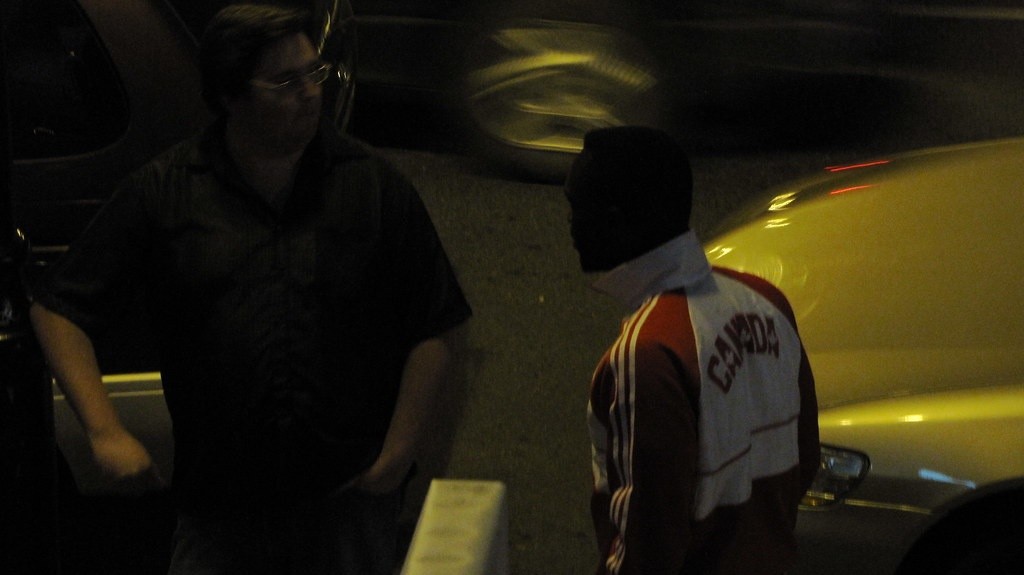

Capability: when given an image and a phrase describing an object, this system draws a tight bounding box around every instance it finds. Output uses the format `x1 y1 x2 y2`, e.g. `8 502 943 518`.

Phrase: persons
27 4 474 575
561 124 824 575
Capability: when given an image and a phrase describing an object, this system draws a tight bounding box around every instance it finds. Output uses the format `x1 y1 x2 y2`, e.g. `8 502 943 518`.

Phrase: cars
700 137 1024 575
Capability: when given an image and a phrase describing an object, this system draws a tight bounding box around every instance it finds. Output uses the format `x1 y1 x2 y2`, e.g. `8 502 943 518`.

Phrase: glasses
247 63 330 95
